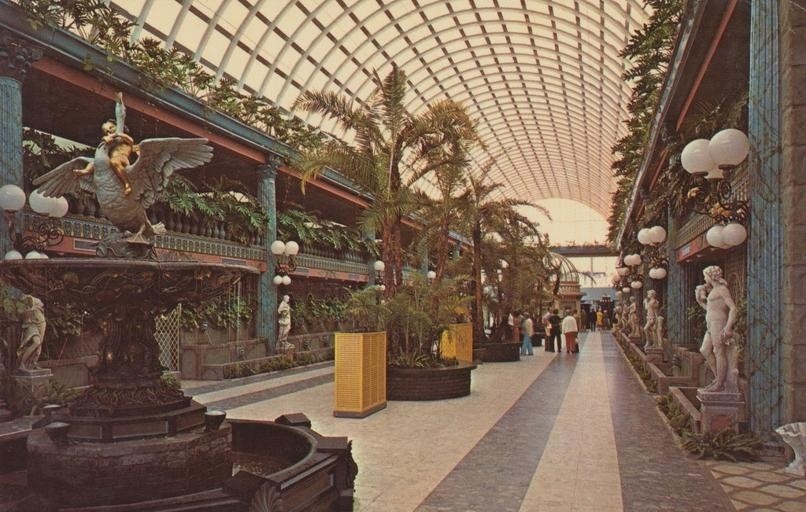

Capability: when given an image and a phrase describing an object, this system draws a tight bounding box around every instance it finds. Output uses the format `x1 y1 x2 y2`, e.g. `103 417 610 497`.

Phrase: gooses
31 91 214 246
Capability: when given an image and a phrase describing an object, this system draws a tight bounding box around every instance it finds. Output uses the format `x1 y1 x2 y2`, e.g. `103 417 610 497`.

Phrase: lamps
681 128 750 250
611 226 670 298
271 240 299 286
0 184 69 259
427 270 436 286
374 260 386 293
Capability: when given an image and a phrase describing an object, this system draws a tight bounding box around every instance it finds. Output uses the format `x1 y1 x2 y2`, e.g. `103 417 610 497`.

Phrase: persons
542 305 610 353
644 289 659 347
73 120 134 197
17 294 46 374
614 298 640 340
508 310 534 356
278 295 291 346
697 266 736 391
